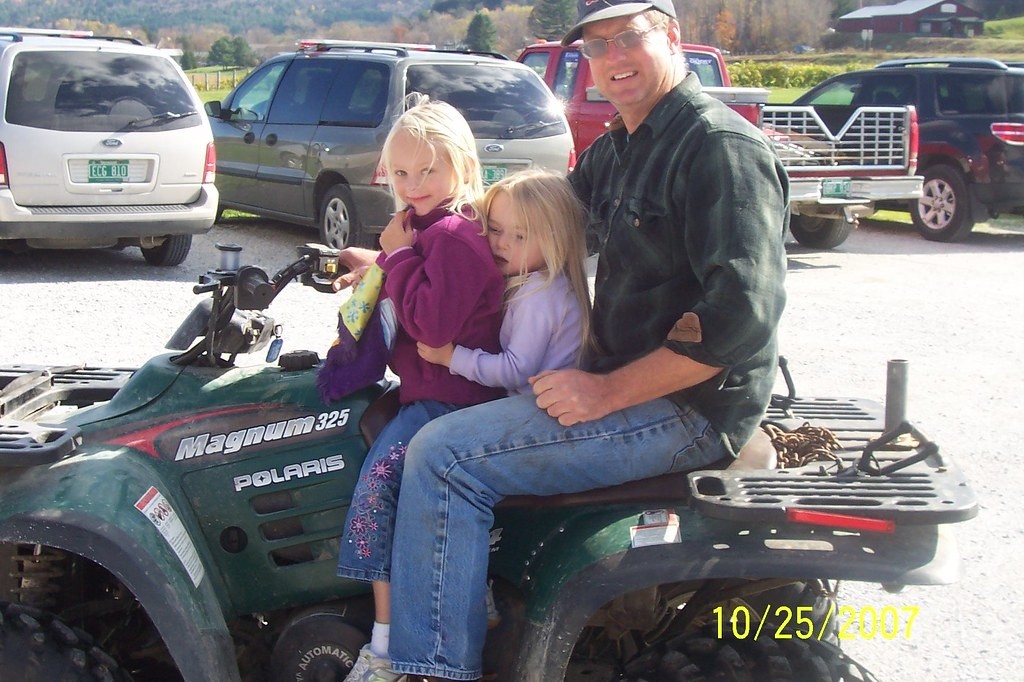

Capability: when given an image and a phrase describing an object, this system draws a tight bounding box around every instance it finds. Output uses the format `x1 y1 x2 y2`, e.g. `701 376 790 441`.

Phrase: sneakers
342 644 412 682
486 579 502 628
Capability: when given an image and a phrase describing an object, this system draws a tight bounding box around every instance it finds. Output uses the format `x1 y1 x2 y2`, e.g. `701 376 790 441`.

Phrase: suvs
204 38 579 252
787 54 1024 242
0 26 219 268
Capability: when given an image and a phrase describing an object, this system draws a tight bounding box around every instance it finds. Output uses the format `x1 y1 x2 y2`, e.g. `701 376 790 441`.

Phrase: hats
561 0 677 46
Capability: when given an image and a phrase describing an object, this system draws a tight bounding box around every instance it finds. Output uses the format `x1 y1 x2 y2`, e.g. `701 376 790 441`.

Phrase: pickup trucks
517 37 926 250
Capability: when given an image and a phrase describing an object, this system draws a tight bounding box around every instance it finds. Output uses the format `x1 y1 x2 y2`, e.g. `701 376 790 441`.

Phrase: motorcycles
2 240 986 682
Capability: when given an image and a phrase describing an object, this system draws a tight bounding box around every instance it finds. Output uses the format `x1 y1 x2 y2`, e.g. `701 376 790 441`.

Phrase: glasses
576 20 669 59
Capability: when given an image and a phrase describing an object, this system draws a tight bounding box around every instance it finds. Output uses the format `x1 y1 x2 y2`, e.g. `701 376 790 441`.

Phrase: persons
415 169 589 628
336 92 505 682
330 0 788 682
